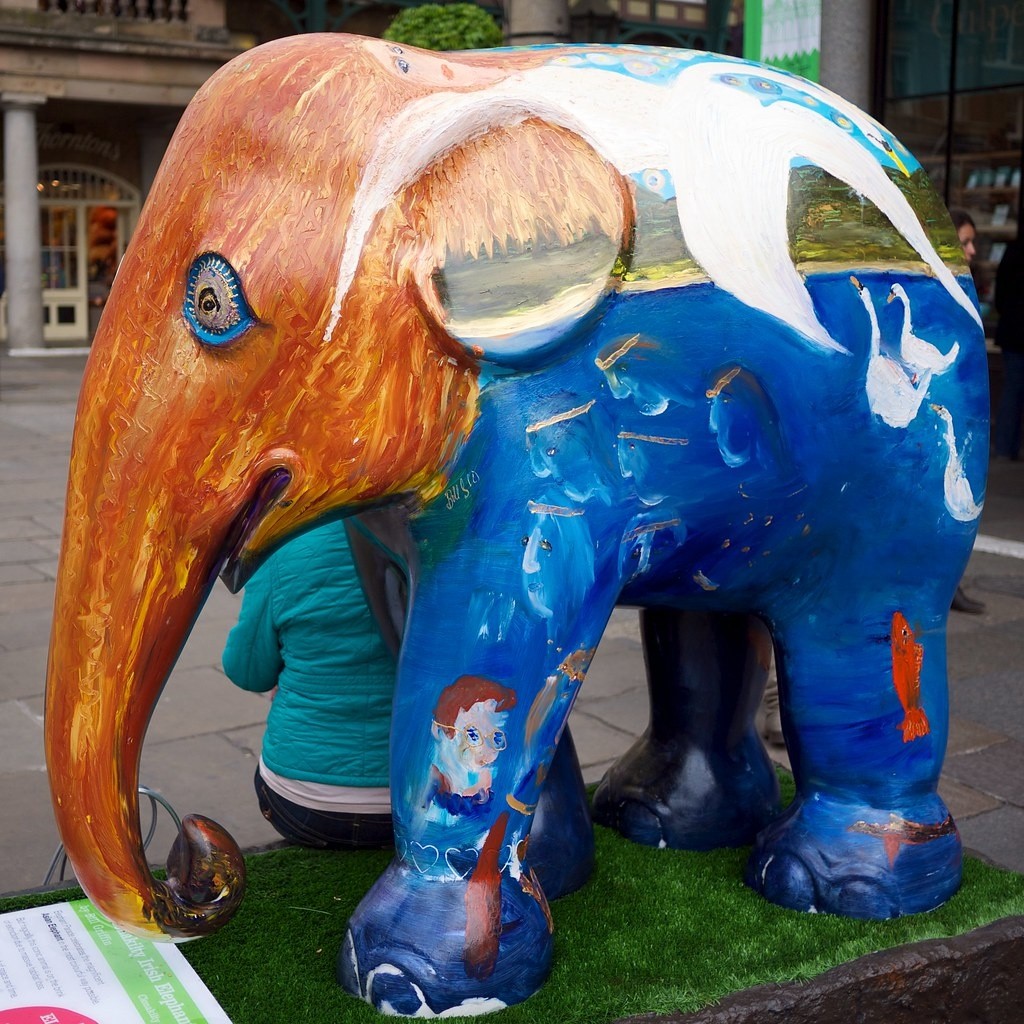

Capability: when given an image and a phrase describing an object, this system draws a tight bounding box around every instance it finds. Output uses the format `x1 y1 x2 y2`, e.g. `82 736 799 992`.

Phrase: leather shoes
950 588 986 614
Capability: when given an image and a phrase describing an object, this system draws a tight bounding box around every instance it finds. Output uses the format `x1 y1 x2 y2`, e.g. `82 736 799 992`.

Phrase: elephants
46 32 994 1019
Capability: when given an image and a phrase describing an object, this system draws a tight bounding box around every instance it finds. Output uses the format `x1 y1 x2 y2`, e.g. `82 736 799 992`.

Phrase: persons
949 207 1002 616
222 518 397 855
